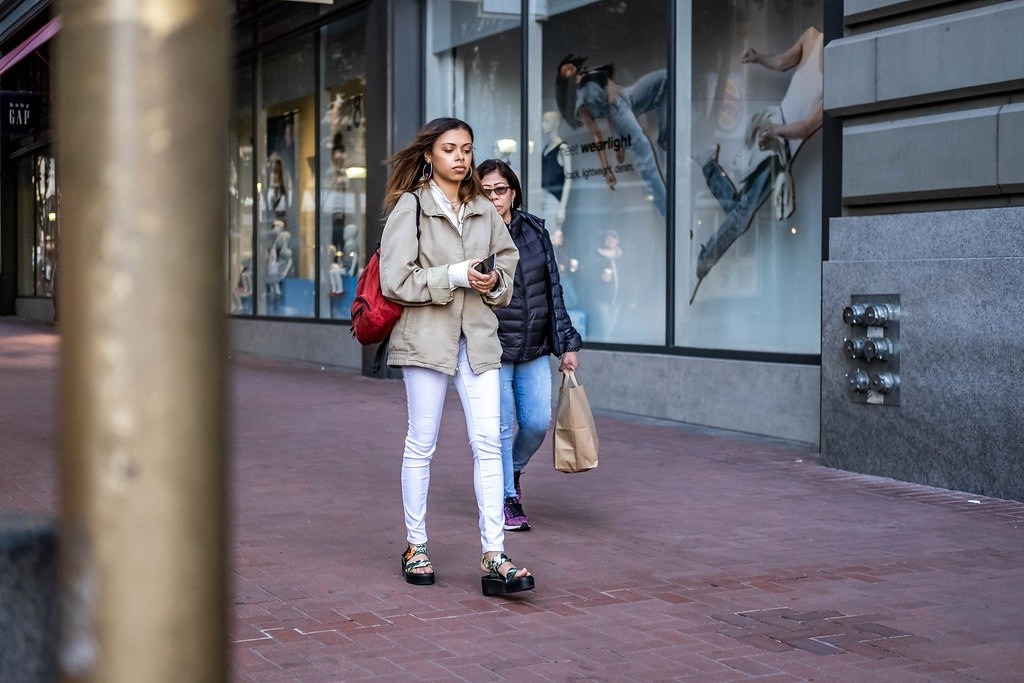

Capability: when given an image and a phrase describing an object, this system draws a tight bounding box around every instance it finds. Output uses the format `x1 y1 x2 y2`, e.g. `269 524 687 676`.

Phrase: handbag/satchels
350 244 404 346
554 369 600 474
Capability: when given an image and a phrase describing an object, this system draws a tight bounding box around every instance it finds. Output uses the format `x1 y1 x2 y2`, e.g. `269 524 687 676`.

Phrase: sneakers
513 471 522 502
504 499 531 530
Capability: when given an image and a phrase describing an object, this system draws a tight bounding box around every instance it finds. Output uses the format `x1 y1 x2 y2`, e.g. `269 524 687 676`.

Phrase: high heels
402 544 436 585
481 553 535 595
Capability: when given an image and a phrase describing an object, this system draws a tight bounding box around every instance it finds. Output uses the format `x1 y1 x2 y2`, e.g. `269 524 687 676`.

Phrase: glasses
484 186 510 196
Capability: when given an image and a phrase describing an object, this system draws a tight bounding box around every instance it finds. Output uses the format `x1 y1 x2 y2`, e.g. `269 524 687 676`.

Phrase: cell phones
474 254 496 275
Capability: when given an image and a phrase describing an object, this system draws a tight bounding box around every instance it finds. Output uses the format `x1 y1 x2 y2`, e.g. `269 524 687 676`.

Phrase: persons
329 224 359 297
266 220 292 299
556 54 670 222
689 27 824 305
476 159 582 530
539 112 571 246
379 117 535 596
232 256 253 312
